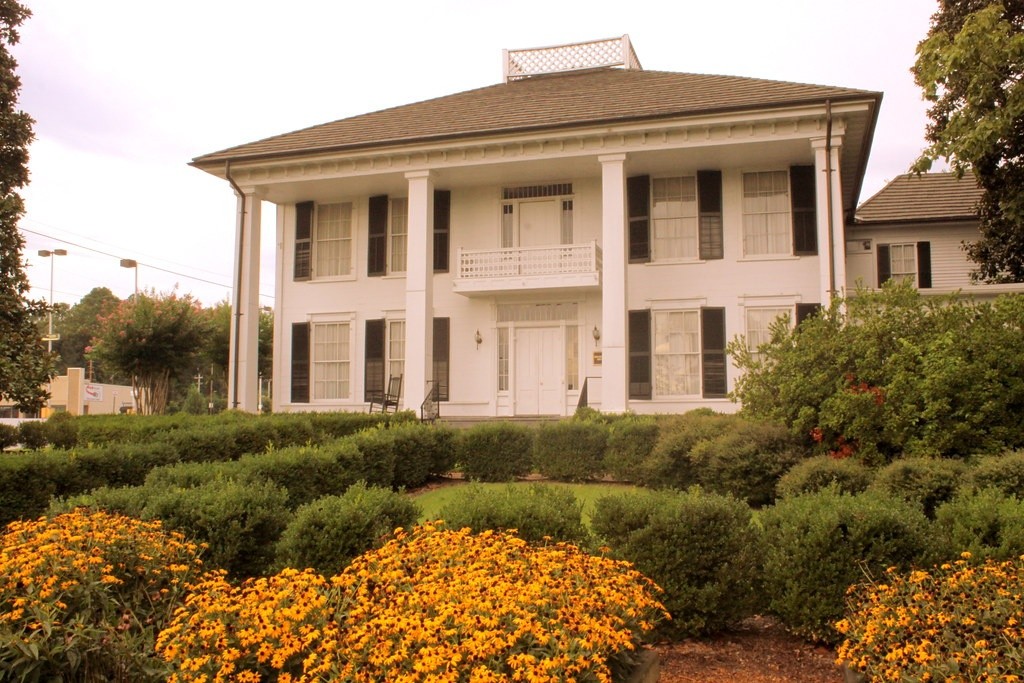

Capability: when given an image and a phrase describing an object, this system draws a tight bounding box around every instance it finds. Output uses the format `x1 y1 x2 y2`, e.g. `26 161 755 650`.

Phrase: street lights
121 259 137 305
38 249 66 352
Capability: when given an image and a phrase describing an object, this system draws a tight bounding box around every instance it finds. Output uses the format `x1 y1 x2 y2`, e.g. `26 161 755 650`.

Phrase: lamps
474 328 481 351
593 325 601 348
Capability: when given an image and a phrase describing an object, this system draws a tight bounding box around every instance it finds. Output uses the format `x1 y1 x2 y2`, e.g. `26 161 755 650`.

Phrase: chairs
369 372 402 414
